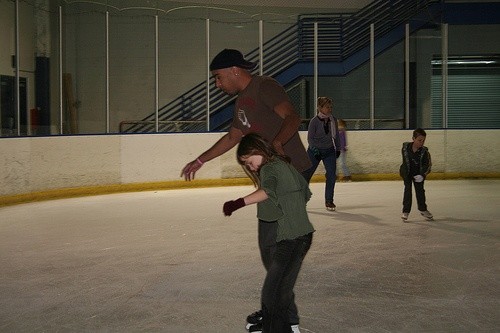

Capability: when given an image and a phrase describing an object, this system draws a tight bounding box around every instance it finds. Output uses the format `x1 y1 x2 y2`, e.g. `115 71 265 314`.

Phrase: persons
325 118 352 181
179 47 316 333
222 134 316 333
399 129 433 221
307 97 341 208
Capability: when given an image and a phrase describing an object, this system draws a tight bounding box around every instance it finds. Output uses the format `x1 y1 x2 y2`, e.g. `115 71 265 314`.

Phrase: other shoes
340 176 352 183
249 322 301 333
246 308 263 325
325 200 335 210
401 213 408 222
418 210 433 221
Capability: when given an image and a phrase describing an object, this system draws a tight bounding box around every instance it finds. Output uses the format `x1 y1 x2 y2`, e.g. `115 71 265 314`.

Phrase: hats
209 48 257 70
316 96 333 111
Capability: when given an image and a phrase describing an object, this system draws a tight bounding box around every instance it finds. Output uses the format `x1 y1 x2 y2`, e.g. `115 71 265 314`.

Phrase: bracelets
196 158 203 166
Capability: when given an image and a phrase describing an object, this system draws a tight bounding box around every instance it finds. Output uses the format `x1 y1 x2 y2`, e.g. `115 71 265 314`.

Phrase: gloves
222 198 246 216
413 175 424 182
